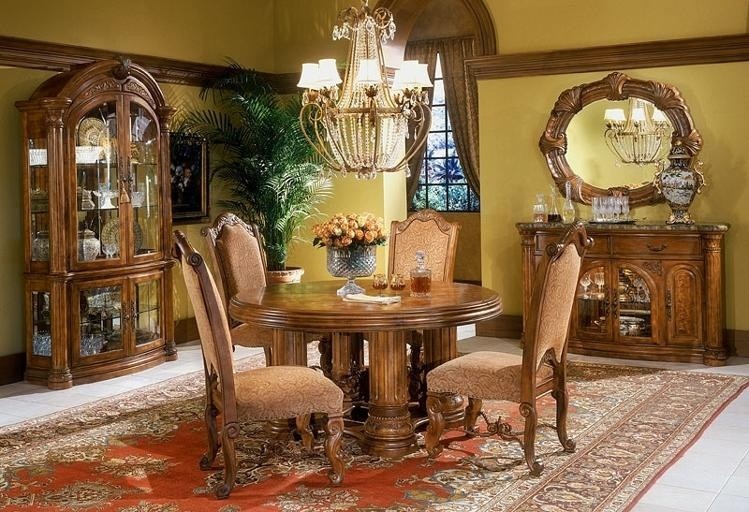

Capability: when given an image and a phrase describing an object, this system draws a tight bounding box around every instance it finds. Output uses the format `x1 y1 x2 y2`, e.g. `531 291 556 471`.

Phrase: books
342 293 401 304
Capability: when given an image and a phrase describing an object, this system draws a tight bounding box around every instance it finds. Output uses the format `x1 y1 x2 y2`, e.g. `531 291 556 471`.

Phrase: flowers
310 212 389 250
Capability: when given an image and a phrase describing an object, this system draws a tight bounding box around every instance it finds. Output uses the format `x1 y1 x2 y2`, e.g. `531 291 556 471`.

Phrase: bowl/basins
618 316 648 337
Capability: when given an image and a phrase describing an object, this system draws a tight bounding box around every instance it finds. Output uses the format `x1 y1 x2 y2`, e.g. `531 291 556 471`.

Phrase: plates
78 118 110 162
100 214 144 260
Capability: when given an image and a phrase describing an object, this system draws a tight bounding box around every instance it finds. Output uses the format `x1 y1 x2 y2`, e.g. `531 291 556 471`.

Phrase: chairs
424 217 597 479
171 227 349 501
387 208 465 401
201 213 326 369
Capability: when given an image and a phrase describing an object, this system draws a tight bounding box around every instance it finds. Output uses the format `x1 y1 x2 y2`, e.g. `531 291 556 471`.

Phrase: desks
221 275 507 460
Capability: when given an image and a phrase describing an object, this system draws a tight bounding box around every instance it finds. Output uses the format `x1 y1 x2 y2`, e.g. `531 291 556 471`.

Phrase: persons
171 163 199 209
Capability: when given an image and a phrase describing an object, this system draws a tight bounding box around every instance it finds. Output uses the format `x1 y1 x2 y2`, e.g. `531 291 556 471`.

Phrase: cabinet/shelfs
513 219 731 369
19 258 180 390
12 52 179 278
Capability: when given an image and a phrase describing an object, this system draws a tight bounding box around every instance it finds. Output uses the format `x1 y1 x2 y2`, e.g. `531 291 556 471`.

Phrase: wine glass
373 274 406 299
580 271 604 301
591 195 630 222
82 184 119 209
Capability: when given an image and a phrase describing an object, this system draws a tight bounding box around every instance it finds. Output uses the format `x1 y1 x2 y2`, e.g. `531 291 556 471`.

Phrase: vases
652 141 709 225
324 244 378 296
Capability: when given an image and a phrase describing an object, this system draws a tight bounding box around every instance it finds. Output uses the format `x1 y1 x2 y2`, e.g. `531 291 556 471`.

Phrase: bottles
82 228 99 261
531 180 576 224
32 230 49 259
409 250 435 299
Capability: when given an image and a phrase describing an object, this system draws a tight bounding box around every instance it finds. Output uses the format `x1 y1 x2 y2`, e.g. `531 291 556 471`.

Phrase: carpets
0 337 749 512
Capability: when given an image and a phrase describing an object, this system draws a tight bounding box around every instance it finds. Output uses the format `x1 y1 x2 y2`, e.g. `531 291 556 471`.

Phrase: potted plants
169 51 341 285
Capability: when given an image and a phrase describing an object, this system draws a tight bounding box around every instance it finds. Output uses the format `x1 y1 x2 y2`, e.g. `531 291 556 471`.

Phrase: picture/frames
168 130 212 224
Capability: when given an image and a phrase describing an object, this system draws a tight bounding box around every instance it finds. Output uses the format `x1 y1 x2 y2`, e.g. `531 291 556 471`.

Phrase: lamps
294 1 435 183
601 94 674 169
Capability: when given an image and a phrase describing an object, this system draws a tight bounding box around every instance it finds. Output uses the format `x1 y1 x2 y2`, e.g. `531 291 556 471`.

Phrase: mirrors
535 69 705 212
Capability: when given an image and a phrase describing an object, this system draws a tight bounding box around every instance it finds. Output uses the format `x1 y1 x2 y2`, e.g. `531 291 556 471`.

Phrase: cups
32 333 51 356
84 286 120 319
80 334 108 356
132 191 145 207
